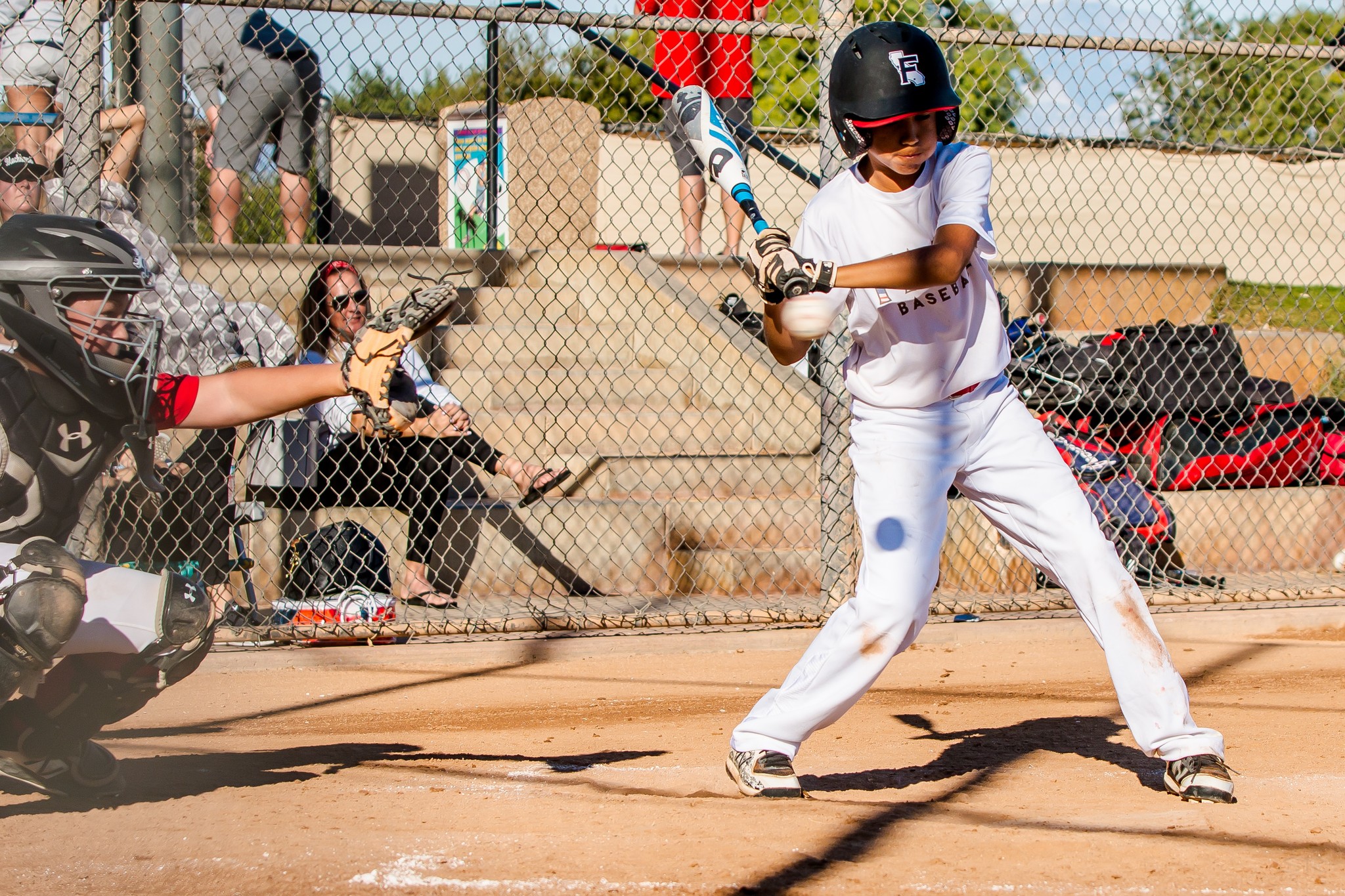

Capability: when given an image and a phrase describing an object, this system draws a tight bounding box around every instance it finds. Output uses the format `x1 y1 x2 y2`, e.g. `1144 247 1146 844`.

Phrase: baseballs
782 295 831 336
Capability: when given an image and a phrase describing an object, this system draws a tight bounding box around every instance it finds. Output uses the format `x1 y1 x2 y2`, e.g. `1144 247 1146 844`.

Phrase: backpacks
1035 417 1177 583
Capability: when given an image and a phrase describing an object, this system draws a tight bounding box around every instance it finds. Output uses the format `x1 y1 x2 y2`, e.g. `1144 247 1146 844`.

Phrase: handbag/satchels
284 520 393 601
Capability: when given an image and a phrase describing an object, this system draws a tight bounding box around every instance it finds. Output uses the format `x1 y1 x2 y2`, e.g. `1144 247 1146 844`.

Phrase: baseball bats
669 86 806 298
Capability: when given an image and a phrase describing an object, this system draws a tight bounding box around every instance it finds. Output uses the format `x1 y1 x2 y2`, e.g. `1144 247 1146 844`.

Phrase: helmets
829 21 962 160
0 210 147 416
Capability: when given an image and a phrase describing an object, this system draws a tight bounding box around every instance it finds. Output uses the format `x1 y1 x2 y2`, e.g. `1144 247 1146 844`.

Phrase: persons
292 258 571 608
723 19 1235 805
0 0 298 377
633 0 777 257
180 0 320 243
0 213 459 797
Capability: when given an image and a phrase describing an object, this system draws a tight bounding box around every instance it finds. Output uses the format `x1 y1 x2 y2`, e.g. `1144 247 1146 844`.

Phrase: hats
0 149 49 175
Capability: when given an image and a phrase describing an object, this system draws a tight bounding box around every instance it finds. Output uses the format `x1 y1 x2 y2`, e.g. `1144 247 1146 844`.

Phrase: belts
948 381 981 400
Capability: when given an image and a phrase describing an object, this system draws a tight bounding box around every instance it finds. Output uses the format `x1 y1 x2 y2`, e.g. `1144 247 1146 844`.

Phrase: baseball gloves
346 283 459 419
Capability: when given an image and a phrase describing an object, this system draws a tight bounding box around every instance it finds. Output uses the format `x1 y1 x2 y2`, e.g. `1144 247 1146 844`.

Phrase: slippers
518 468 571 508
401 589 457 607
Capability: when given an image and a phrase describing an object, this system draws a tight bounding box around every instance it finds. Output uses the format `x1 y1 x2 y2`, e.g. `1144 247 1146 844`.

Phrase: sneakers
1163 754 1241 804
216 600 283 647
725 749 801 797
0 698 119 798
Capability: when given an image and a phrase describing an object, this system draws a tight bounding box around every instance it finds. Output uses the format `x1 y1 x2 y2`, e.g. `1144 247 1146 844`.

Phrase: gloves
747 228 790 305
766 248 838 294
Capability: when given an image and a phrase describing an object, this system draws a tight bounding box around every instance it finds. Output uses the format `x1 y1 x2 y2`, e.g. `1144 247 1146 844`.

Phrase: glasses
328 289 368 312
